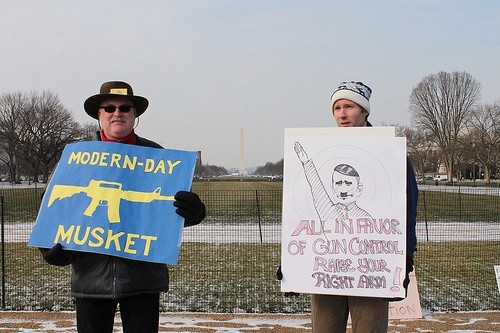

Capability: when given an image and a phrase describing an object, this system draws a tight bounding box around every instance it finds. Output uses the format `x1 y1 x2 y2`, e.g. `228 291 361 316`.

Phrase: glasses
98 104 136 113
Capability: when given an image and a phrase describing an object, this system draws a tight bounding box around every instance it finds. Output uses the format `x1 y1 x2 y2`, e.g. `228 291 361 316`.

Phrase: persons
277 81 419 333
38 81 206 333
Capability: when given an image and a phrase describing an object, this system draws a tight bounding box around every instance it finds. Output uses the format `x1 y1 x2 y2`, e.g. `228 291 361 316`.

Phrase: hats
84 81 149 120
330 80 372 117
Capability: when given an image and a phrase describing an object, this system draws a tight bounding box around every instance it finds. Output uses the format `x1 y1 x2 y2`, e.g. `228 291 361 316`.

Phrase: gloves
173 191 206 227
38 243 76 266
277 265 300 297
383 273 410 302
